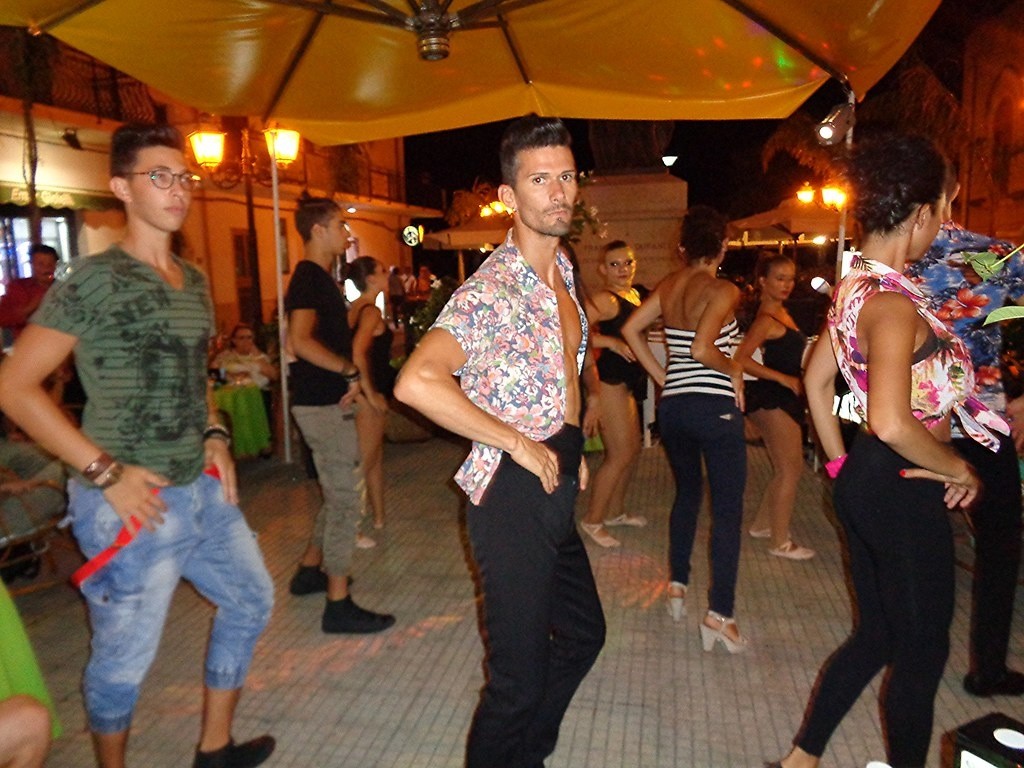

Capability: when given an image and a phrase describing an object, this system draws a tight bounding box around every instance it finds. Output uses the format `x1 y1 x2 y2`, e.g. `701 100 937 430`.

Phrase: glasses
111 168 201 192
608 259 634 267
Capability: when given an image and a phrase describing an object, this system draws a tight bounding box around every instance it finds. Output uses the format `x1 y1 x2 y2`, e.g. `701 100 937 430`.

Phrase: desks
211 380 271 457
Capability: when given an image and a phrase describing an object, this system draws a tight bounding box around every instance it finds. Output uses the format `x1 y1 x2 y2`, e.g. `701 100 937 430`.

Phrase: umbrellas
0 0 940 146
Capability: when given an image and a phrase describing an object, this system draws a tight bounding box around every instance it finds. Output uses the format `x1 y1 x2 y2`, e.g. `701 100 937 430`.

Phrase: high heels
698 610 751 655
664 580 687 622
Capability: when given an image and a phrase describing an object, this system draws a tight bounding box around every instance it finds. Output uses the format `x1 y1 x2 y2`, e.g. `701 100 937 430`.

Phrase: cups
223 384 234 392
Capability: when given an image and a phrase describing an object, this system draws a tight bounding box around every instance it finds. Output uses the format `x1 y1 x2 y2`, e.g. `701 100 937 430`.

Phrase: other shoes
318 610 394 634
767 543 817 560
748 522 790 540
371 518 384 530
579 517 621 549
290 571 353 592
603 514 647 528
963 668 1024 698
356 535 376 549
193 735 276 768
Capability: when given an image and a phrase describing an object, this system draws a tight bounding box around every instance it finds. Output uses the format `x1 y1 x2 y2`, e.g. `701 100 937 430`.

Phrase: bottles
210 373 217 391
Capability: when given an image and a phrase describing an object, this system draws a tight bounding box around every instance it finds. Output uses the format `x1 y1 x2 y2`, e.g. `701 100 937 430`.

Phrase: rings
1007 416 1015 423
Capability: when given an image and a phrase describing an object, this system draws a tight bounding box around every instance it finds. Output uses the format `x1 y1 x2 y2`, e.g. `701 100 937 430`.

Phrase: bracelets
825 453 848 479
99 462 126 491
203 424 232 443
83 451 113 481
347 367 361 383
340 357 350 376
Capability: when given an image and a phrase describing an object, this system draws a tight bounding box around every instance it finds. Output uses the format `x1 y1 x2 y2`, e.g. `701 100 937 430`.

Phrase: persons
0 577 60 768
282 197 397 635
0 242 70 380
387 264 446 331
765 128 1024 768
537 208 816 655
0 122 275 768
339 256 393 549
212 301 273 395
392 128 607 768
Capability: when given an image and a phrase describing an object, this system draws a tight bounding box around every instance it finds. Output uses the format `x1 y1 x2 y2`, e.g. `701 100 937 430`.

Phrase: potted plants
385 359 442 442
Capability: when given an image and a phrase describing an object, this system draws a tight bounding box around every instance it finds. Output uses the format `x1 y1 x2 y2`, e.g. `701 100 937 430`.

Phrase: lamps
62 127 82 150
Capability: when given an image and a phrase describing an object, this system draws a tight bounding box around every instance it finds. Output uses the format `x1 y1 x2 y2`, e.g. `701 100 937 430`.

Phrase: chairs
0 470 86 597
